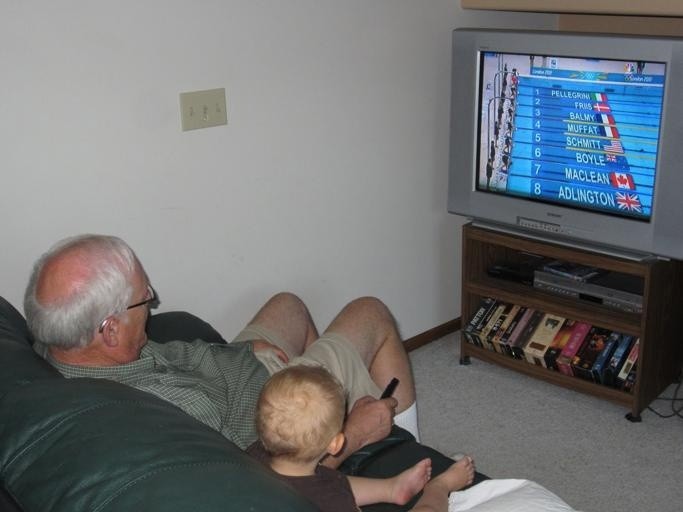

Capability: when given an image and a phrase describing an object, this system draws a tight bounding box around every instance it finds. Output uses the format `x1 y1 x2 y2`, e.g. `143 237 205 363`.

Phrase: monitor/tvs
446 29 683 263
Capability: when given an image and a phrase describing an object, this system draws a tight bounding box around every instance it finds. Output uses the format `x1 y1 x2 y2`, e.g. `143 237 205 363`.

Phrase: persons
22 234 421 458
484 64 517 190
241 365 476 510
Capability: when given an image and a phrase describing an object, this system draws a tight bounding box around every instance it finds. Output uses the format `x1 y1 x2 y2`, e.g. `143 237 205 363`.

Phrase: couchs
0 290 496 512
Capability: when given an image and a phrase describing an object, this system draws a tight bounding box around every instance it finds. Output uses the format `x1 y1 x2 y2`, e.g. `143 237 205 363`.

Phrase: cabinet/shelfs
457 222 681 423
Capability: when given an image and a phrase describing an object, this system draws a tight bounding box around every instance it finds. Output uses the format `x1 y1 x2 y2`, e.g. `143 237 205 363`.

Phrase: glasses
98 285 159 333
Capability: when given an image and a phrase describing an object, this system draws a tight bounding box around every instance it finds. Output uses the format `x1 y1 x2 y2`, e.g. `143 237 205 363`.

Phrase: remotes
379 375 400 401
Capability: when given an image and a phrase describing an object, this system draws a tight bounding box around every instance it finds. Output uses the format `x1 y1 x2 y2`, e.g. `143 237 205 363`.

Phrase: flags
590 91 642 214
